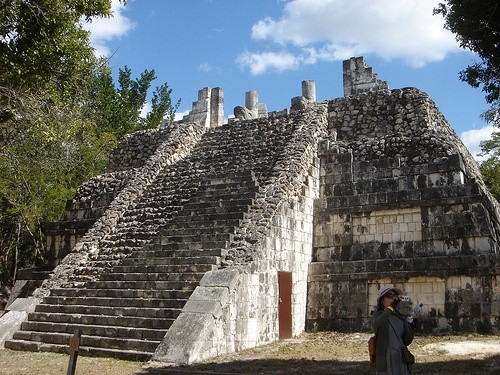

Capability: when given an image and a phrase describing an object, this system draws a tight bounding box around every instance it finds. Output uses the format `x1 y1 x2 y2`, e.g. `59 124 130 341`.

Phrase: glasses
385 292 398 299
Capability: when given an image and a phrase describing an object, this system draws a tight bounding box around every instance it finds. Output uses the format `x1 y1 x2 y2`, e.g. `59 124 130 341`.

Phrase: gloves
390 298 401 309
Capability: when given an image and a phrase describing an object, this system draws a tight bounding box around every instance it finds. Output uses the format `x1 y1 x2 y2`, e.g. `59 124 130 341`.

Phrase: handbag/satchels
402 346 414 364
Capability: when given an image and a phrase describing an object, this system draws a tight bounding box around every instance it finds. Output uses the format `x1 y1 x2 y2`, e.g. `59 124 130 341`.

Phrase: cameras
399 295 409 301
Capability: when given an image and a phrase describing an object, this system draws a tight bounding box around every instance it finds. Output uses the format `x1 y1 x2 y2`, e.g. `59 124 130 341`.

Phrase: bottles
406 303 423 323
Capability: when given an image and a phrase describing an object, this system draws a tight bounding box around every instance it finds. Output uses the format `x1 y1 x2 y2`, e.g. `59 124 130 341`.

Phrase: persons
371 286 422 375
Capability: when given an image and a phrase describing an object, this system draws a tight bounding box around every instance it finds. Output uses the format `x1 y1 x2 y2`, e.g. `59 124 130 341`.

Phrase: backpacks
368 336 377 368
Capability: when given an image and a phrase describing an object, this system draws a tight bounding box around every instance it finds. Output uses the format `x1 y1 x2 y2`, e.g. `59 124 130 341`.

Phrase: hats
377 286 403 305
